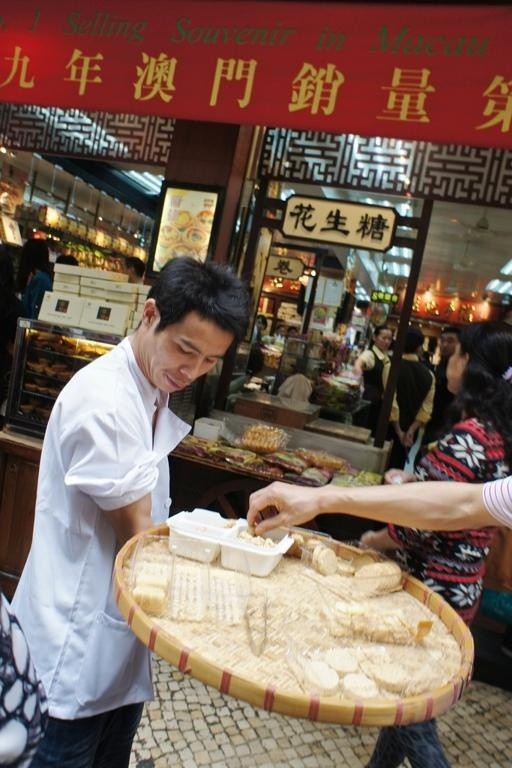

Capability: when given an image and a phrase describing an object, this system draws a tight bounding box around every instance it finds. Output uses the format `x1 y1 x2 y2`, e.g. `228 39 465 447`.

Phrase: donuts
159 210 213 258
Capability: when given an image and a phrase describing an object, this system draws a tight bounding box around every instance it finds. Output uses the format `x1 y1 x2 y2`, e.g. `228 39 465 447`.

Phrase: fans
449 204 511 237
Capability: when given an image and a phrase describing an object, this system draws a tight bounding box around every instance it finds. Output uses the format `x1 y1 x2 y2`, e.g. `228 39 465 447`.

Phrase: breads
132 560 171 612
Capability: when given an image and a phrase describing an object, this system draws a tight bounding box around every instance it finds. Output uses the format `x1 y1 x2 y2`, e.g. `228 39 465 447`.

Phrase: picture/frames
144 179 226 279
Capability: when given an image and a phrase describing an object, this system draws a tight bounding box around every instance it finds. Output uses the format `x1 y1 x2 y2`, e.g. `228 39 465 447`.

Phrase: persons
11 255 260 767
2 239 78 392
352 316 511 768
119 255 144 283
256 315 297 338
353 327 463 472
243 471 511 540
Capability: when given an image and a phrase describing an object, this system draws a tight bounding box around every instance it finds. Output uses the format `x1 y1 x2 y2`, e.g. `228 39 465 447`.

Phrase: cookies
286 532 434 699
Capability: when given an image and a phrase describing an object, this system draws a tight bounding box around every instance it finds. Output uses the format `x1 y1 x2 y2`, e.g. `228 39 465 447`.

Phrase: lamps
0 145 143 240
